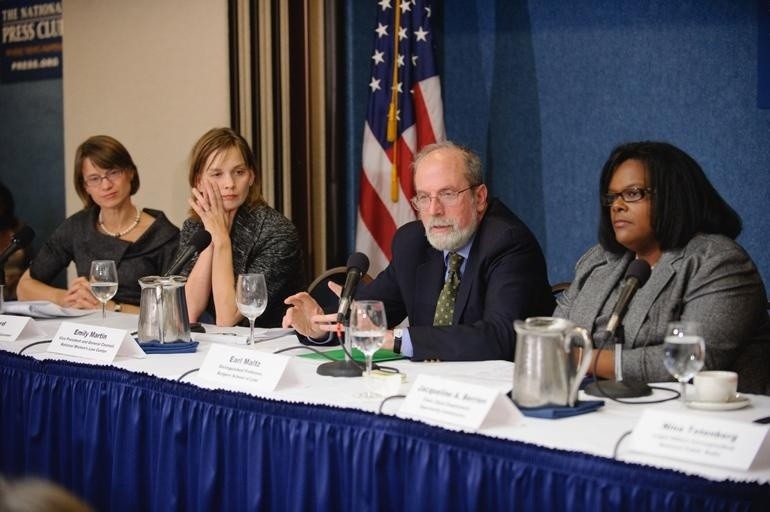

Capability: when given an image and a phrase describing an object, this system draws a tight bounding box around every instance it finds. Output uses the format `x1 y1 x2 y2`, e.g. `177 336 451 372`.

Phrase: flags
354 0 447 329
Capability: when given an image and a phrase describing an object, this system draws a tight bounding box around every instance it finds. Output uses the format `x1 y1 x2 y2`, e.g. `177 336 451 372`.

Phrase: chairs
306 266 374 315
548 283 572 293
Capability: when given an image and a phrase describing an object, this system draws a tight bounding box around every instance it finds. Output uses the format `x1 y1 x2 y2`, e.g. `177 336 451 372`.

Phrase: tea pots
512 317 593 407
137 274 191 344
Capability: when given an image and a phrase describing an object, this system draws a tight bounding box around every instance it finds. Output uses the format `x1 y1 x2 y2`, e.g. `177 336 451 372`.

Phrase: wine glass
89 260 118 319
349 300 387 402
235 273 268 350
663 322 705 405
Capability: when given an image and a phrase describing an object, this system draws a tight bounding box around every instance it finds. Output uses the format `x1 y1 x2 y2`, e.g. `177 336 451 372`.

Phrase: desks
0 300 770 511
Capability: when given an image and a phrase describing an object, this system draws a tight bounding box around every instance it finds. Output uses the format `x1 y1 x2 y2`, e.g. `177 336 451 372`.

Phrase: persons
15 134 181 315
549 140 770 397
281 140 557 363
0 185 31 302
158 126 306 328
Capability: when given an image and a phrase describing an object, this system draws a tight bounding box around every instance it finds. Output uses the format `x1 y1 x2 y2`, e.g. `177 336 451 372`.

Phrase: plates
685 397 751 411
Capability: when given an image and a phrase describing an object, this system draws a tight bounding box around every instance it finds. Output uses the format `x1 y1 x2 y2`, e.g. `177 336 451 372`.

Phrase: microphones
603 258 652 342
0 225 36 266
162 229 212 276
336 252 370 322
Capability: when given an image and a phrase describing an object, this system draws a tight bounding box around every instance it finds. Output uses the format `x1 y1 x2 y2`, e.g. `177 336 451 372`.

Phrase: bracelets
575 347 583 374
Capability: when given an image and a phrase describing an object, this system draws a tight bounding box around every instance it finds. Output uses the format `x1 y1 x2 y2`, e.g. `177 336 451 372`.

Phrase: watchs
115 301 122 312
393 327 402 353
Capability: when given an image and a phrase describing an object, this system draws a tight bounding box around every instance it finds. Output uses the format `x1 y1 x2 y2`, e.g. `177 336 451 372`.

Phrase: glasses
77 163 127 192
604 183 658 205
411 185 479 212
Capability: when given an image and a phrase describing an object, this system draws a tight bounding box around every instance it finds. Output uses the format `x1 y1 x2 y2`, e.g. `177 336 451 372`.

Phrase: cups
694 371 737 403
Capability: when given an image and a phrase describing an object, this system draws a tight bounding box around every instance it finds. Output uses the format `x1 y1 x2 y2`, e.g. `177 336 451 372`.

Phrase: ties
432 253 467 332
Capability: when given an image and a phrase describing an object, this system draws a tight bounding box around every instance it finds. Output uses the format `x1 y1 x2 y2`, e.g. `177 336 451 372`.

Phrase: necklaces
635 252 661 271
98 207 140 239
